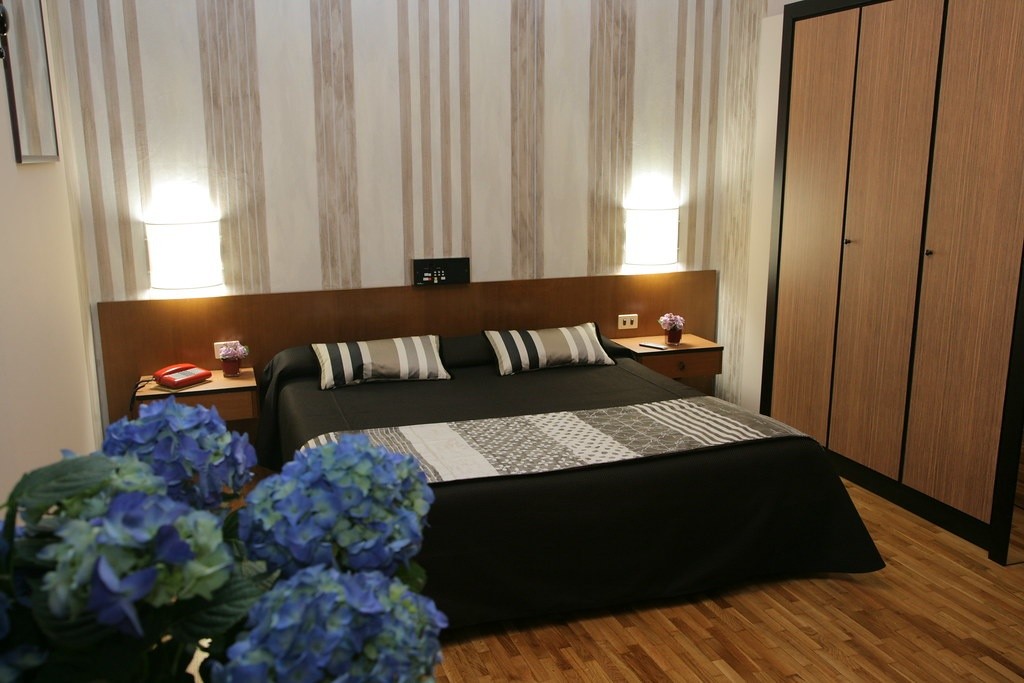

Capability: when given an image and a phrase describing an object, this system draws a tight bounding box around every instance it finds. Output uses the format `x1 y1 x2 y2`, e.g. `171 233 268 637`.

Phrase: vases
219 359 241 379
664 328 683 346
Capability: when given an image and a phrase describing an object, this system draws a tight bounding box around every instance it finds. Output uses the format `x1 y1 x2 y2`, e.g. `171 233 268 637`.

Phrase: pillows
481 321 618 377
310 333 452 391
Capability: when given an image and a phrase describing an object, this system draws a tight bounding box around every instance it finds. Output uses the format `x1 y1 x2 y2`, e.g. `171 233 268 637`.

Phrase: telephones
154 364 212 388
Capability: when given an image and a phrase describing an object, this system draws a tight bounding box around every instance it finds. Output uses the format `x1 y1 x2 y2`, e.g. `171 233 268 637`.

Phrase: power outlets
213 341 226 360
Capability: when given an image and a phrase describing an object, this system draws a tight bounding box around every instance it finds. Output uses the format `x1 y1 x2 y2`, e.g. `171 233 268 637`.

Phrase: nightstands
609 333 724 399
134 367 260 450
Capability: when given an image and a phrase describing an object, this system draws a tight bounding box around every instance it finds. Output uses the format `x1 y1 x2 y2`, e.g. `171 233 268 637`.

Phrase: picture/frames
0 0 60 166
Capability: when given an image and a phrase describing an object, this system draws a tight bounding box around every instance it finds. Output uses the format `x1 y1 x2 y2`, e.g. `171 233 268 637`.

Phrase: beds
268 332 831 647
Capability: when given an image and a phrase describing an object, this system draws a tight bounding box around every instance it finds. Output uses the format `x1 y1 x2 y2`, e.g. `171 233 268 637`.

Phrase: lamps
129 176 233 300
619 175 681 273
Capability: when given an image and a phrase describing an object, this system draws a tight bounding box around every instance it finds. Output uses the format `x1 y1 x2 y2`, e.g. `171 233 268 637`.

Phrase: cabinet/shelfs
767 0 1024 568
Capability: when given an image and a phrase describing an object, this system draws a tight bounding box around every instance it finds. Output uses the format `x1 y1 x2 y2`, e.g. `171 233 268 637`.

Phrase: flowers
657 312 687 331
0 395 450 683
217 342 250 361
